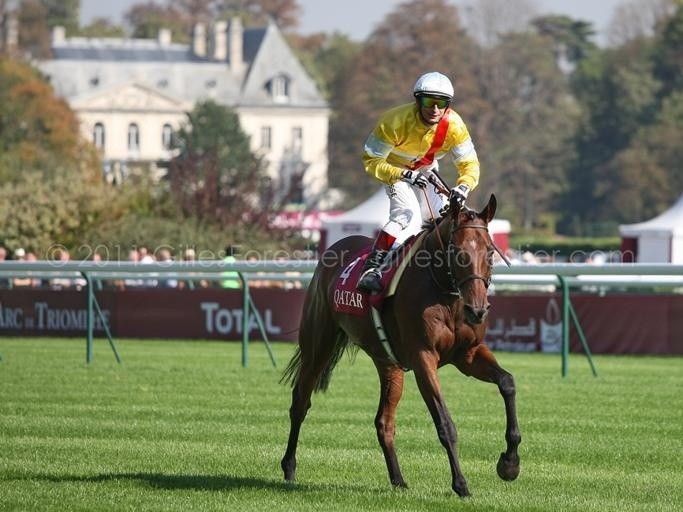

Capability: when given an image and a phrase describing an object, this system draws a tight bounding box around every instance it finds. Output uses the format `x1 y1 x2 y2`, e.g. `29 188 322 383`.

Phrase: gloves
400 170 429 189
447 183 471 211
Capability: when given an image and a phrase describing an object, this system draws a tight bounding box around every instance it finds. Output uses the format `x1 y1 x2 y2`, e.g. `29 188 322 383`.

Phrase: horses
277 190 522 499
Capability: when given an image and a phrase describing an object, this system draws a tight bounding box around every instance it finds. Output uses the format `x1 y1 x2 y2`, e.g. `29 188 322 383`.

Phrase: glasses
418 96 450 109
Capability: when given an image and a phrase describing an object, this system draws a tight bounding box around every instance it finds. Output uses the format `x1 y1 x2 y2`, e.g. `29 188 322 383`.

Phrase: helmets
414 71 455 102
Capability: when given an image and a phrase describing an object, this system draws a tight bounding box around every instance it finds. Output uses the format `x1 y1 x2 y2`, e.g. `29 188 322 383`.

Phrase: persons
1 243 683 293
346 71 480 296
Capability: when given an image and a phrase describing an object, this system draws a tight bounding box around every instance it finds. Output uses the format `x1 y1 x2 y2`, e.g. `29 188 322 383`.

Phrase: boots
358 246 397 291
